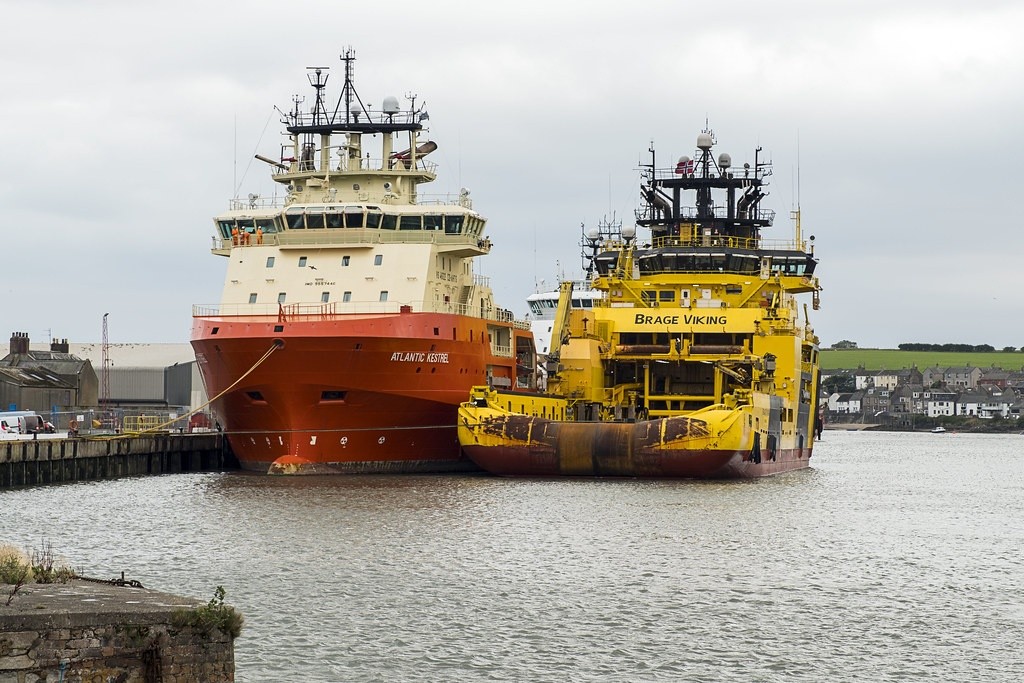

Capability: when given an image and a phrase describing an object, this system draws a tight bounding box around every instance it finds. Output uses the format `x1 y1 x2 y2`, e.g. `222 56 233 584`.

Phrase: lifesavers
70 420 78 430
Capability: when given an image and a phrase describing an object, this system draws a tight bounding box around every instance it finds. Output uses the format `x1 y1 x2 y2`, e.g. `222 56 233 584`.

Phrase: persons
232 225 251 245
256 226 263 245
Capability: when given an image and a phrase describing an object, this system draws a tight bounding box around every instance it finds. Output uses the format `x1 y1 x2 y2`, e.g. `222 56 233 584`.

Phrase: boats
526 257 608 392
457 118 826 484
190 44 537 477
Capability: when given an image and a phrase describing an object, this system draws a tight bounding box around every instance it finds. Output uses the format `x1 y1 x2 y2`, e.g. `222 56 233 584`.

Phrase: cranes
100 312 117 429
